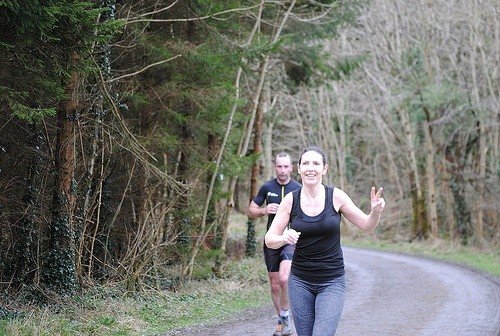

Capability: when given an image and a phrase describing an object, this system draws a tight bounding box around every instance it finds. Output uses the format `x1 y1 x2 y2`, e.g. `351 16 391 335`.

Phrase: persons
263 147 386 336
247 151 303 335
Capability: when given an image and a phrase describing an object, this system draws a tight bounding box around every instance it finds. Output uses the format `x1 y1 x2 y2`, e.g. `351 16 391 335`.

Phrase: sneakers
281 317 293 335
273 320 281 336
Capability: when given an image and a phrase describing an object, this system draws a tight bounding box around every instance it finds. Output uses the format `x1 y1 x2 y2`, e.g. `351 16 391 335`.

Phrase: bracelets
369 210 382 216
